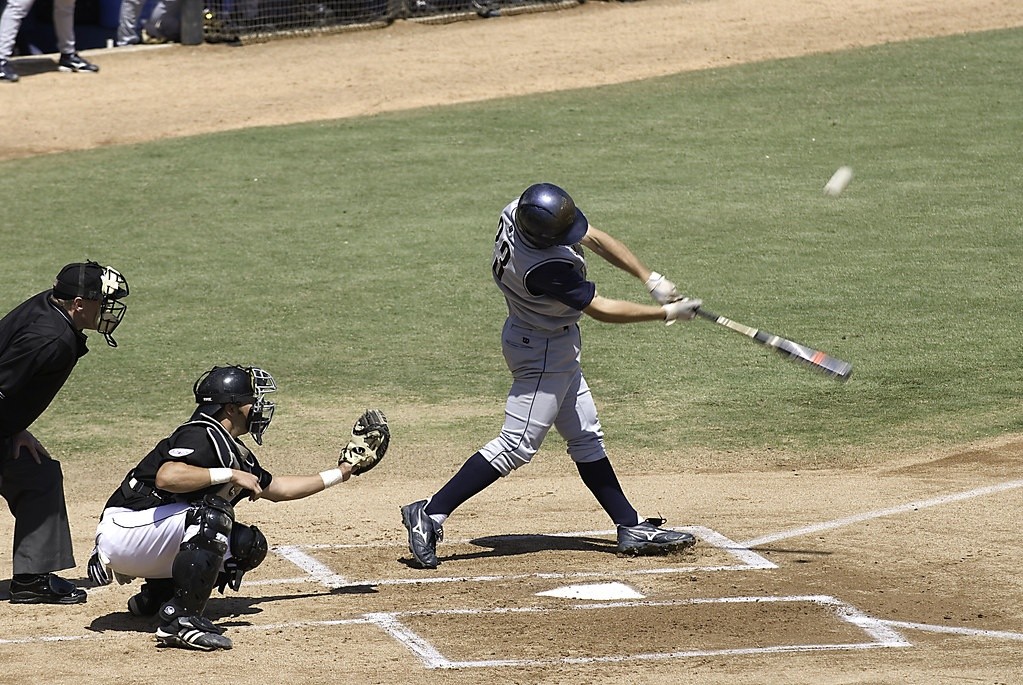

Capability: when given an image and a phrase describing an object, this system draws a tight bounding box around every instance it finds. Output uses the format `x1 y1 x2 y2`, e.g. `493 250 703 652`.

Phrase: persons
117 0 177 46
0 0 98 82
221 0 272 33
400 183 702 567
0 260 130 604
87 364 391 651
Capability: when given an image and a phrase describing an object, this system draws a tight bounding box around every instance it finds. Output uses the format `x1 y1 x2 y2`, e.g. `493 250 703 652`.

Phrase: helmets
518 182 588 248
189 366 260 420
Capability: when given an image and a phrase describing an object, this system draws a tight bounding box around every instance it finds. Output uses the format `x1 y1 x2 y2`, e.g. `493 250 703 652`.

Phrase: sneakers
57 53 98 73
616 512 696 557
129 593 161 616
401 500 445 569
156 620 213 650
9 571 87 604
0 57 19 82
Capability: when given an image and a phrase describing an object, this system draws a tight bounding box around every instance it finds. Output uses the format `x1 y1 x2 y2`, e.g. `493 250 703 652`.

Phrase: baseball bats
695 306 853 383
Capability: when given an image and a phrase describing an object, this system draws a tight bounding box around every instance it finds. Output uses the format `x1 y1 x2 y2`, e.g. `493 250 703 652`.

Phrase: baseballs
823 166 854 199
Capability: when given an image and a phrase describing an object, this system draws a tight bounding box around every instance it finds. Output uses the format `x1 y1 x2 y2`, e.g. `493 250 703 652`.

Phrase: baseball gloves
338 409 391 476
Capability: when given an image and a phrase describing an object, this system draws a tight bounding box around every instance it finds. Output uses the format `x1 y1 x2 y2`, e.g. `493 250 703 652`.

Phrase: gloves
88 549 114 585
643 271 680 306
663 297 702 322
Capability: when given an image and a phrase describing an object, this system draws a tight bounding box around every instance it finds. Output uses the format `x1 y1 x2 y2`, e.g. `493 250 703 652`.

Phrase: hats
54 260 104 299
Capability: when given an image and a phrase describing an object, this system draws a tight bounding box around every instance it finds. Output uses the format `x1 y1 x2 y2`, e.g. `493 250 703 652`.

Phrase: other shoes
141 30 167 43
116 33 140 46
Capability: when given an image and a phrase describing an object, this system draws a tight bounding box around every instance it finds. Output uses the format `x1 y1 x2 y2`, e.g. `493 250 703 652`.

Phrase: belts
563 325 569 331
